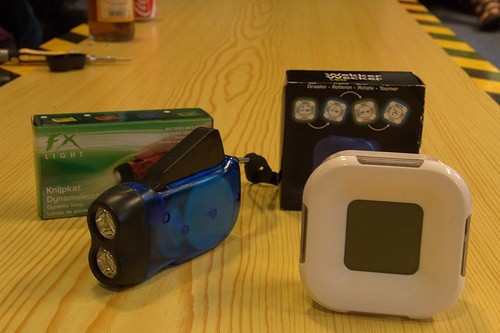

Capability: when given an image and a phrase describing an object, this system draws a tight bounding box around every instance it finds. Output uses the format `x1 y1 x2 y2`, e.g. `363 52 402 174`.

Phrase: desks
1 0 500 333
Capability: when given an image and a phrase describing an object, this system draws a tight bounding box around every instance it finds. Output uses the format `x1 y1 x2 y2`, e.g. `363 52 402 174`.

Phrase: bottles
87 0 135 42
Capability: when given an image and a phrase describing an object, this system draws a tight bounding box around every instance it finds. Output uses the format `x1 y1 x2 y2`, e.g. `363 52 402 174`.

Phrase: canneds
87 0 156 41
